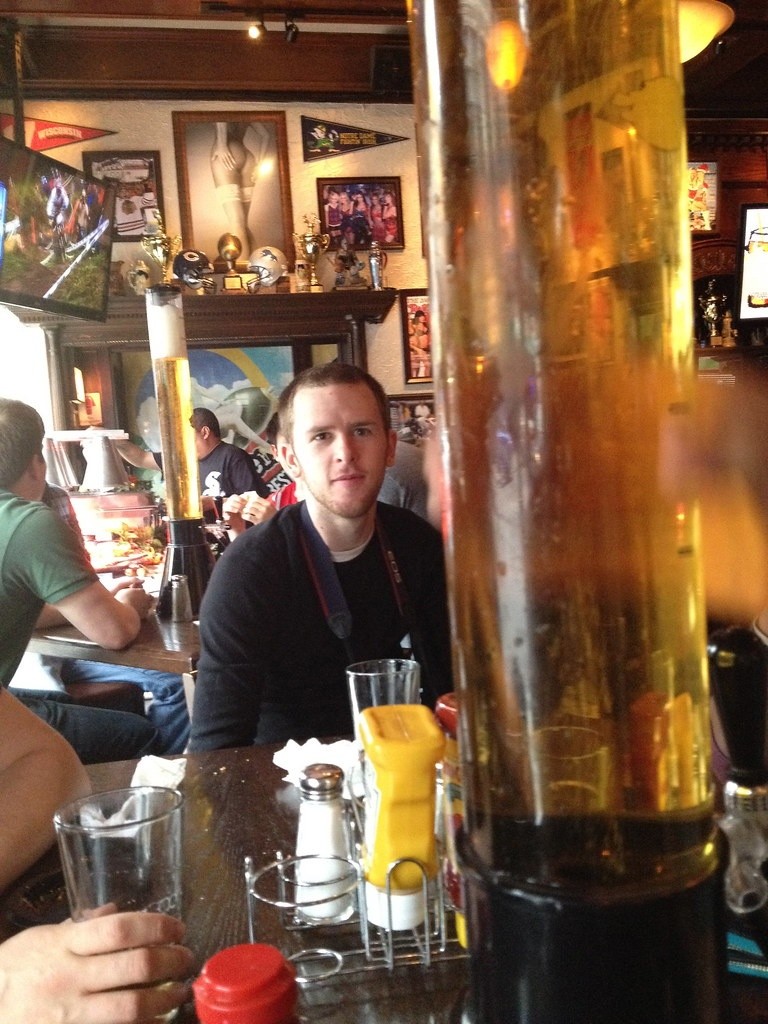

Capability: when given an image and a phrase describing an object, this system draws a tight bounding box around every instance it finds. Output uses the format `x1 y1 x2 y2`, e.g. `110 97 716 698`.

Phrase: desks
0 732 463 1024
4 285 398 434
20 528 235 724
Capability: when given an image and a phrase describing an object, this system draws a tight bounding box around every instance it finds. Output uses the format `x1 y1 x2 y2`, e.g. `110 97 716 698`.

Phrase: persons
187 364 455 754
8 481 192 755
79 408 267 499
323 185 398 245
408 309 428 355
211 121 270 260
41 173 104 260
708 603 768 776
0 679 195 1024
0 398 161 766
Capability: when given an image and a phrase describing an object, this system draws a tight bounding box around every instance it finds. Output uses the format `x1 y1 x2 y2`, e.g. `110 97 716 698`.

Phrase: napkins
268 738 370 805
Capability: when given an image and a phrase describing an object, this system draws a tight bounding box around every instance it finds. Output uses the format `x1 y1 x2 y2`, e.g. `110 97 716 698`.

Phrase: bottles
170 574 194 623
356 691 469 951
193 943 299 1024
292 763 357 926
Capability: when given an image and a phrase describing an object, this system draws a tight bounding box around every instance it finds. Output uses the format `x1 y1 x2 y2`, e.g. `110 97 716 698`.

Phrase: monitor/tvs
0 135 115 324
735 203 768 326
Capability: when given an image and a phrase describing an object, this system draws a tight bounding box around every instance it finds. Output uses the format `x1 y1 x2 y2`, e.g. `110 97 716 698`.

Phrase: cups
127 260 152 296
295 260 310 290
345 658 421 798
51 784 186 1022
522 727 611 813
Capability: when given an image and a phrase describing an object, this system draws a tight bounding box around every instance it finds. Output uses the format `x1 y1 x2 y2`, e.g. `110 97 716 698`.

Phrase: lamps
283 18 298 44
674 0 735 64
248 16 267 42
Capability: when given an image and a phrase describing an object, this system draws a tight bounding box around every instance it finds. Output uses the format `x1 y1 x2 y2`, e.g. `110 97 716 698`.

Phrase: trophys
698 281 727 345
293 213 330 292
141 209 182 285
218 233 242 289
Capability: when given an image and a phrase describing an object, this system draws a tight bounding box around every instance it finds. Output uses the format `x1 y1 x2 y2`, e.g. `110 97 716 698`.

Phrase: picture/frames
688 152 724 235
81 150 167 245
398 288 434 386
316 176 406 251
171 109 297 275
